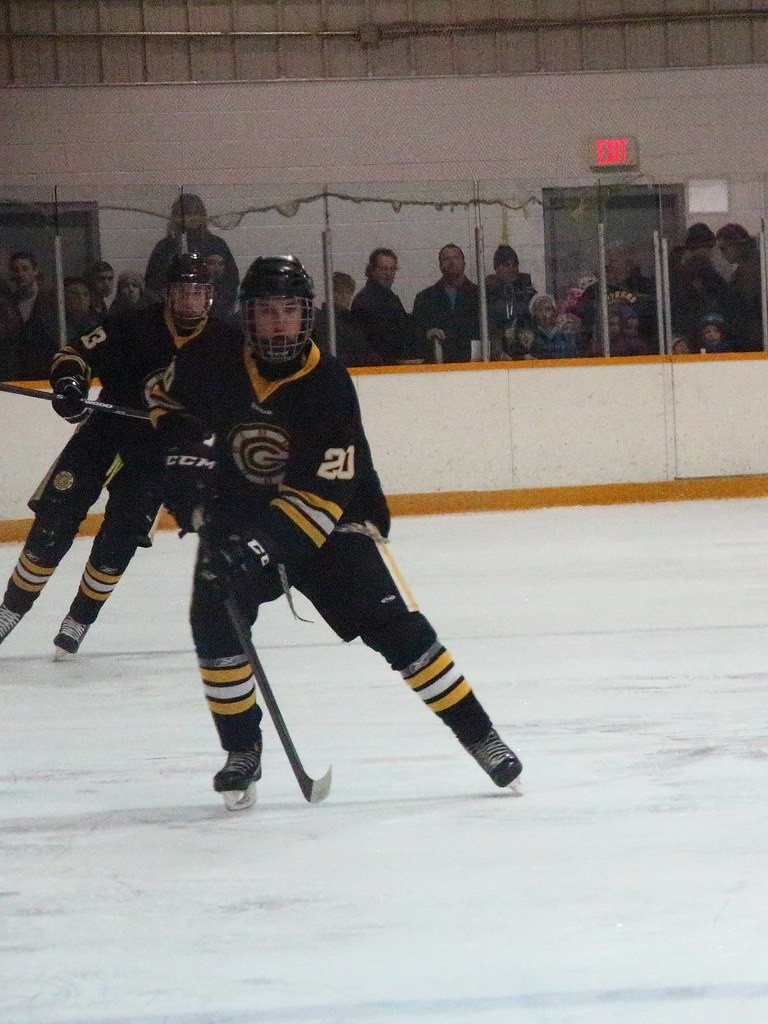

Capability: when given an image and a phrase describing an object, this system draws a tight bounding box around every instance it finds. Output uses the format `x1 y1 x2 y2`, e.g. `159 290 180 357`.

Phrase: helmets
166 254 215 329
241 254 316 367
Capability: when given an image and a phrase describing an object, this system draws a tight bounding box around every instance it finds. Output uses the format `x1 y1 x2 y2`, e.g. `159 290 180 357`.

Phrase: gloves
51 378 91 422
202 525 285 620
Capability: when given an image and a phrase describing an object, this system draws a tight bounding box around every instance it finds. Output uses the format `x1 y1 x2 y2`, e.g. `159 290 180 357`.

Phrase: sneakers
213 744 265 812
52 611 90 664
461 723 520 792
0 602 24 645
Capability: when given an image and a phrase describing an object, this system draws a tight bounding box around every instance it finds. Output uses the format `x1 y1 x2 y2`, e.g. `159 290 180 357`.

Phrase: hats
687 223 716 247
119 271 145 296
528 294 557 313
699 313 725 330
494 245 518 267
64 278 91 295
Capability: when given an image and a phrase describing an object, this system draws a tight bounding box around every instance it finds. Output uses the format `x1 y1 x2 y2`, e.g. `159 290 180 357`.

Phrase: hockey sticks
0 376 156 421
194 507 335 802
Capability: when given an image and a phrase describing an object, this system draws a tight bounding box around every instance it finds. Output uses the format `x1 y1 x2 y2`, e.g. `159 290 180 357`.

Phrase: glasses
376 264 398 274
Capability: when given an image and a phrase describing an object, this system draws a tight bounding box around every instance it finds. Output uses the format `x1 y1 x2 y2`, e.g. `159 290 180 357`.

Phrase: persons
161 254 522 811
505 222 763 358
0 254 226 661
314 271 368 368
0 253 113 381
145 194 239 310
352 248 422 365
110 269 159 312
486 246 539 362
412 245 481 362
